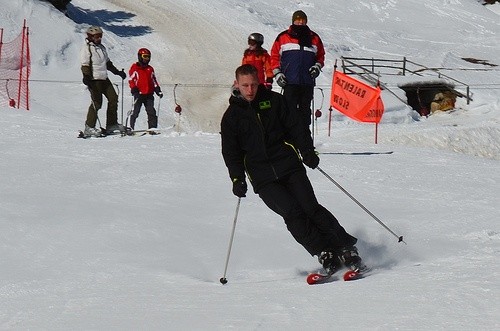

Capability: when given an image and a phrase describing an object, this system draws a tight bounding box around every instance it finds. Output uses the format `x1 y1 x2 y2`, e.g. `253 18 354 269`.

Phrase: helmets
248 33 264 45
87 25 104 35
138 48 151 55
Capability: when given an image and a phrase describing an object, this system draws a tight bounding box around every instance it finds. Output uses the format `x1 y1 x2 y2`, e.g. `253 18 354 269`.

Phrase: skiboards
306 262 366 285
76 123 177 138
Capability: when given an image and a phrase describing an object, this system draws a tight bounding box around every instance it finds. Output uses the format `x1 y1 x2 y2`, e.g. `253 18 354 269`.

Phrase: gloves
309 63 323 78
302 149 320 169
133 91 143 102
232 178 248 198
154 87 164 98
83 75 93 85
272 69 287 87
115 71 126 80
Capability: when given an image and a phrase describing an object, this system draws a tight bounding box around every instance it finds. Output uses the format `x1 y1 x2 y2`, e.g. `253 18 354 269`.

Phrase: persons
80 26 131 136
126 48 162 135
242 32 274 89
220 65 367 272
270 10 326 154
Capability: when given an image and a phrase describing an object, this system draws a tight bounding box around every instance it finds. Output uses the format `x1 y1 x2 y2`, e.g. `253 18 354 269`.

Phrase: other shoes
318 246 367 275
149 128 161 135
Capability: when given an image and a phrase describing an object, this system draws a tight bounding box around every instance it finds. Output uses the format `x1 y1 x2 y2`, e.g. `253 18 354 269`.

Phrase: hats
292 10 307 21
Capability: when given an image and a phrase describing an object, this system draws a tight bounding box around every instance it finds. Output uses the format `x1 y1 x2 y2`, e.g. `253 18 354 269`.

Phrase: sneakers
84 124 104 136
106 123 126 134
